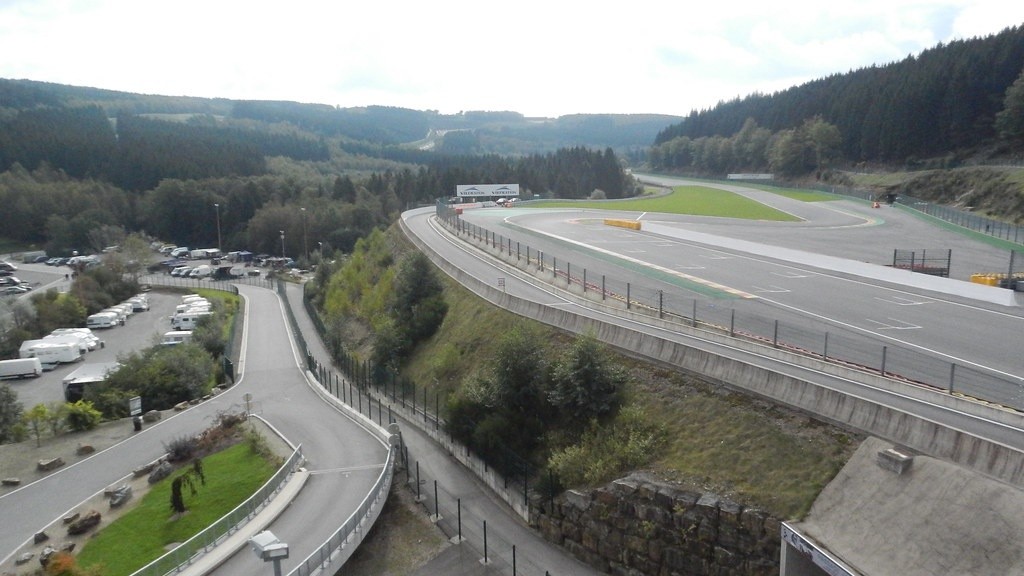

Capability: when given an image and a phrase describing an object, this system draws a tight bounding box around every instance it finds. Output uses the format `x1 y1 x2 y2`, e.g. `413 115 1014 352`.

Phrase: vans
0 328 99 378
162 294 216 345
87 293 148 329
151 241 220 260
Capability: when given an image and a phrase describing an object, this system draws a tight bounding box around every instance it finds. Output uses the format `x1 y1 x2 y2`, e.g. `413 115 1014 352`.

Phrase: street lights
281 230 285 265
301 208 308 261
214 204 221 250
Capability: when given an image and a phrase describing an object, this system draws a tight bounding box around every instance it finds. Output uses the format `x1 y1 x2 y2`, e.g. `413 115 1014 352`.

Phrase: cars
33 255 101 271
496 198 521 205
0 261 32 293
147 260 260 280
